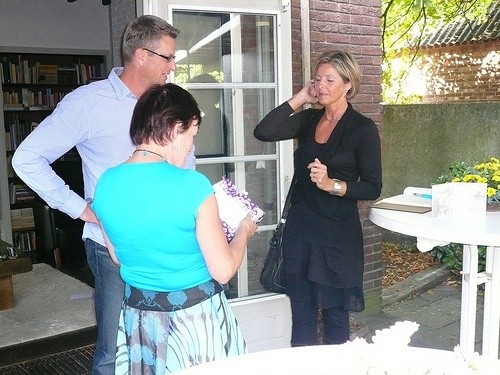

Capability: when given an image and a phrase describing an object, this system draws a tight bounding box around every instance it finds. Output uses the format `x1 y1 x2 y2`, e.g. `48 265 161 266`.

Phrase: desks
173 344 500 375
33 160 84 270
0 239 33 310
369 195 500 360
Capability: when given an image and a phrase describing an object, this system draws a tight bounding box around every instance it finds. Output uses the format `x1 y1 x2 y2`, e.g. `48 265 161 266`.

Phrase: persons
254 51 383 350
12 16 197 375
92 82 259 375
188 73 237 185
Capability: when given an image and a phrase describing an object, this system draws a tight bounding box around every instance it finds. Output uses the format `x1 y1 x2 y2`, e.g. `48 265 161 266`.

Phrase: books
0 54 102 252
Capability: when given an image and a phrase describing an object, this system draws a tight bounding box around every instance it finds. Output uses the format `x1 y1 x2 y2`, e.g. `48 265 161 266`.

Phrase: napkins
339 320 419 375
454 344 485 375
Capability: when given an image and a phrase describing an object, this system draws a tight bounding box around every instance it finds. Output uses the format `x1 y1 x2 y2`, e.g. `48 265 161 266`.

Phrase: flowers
432 156 500 197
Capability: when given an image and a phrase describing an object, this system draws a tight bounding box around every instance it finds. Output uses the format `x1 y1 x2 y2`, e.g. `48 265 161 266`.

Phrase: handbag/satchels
259 222 289 296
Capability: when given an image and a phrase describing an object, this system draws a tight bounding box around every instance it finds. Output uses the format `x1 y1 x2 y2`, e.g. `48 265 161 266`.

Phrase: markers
413 193 432 199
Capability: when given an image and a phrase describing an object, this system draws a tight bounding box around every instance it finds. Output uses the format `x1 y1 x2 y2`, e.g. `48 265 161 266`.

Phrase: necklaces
131 149 166 162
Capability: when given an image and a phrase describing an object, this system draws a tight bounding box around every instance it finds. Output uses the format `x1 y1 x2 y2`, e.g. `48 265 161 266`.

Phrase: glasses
142 47 177 63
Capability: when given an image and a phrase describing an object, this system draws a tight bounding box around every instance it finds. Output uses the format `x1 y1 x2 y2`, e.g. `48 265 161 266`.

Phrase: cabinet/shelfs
0 46 112 262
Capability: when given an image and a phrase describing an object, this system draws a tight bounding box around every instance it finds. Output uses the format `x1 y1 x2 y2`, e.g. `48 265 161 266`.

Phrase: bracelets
329 178 343 195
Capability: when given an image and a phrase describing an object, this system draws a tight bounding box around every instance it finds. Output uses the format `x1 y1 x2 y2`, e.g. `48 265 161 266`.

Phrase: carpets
0 262 98 366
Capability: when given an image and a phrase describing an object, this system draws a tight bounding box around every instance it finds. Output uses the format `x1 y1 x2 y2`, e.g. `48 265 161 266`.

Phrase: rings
314 172 316 178
